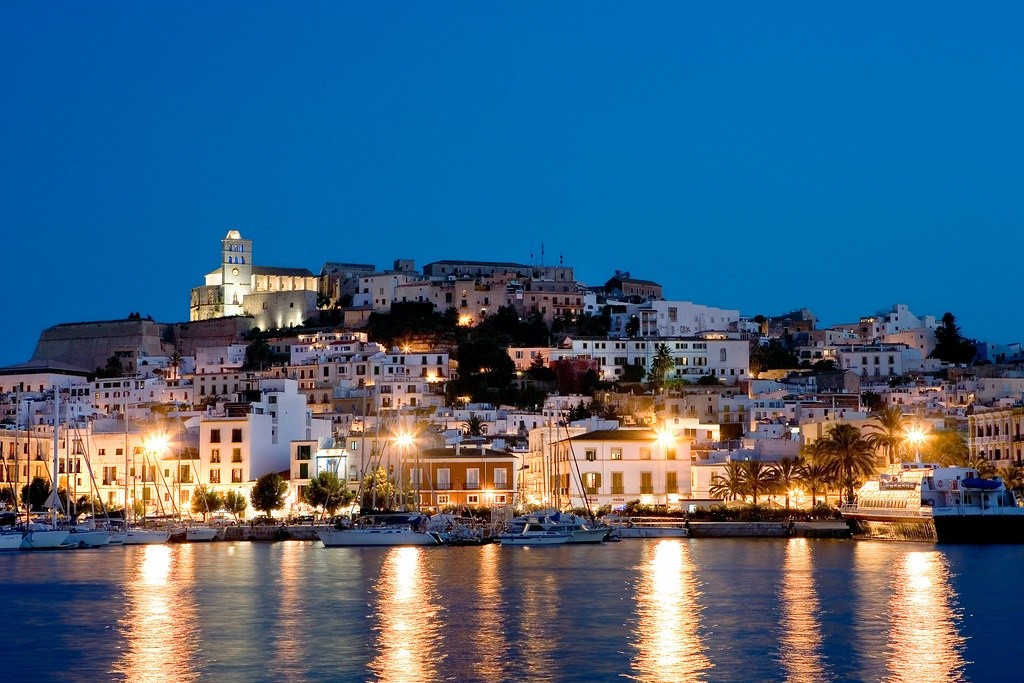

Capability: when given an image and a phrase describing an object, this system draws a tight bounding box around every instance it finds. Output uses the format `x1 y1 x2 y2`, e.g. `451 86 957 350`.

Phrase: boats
841 449 1024 544
603 516 689 537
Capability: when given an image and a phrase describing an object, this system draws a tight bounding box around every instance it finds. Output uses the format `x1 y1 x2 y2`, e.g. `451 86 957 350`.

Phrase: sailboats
494 401 613 546
315 378 484 545
0 385 217 550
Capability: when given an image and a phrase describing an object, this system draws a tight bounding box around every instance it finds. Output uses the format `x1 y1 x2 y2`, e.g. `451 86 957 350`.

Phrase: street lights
790 489 803 509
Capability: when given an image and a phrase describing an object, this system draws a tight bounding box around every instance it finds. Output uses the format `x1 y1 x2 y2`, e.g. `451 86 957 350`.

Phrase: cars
253 515 279 526
211 516 236 527
